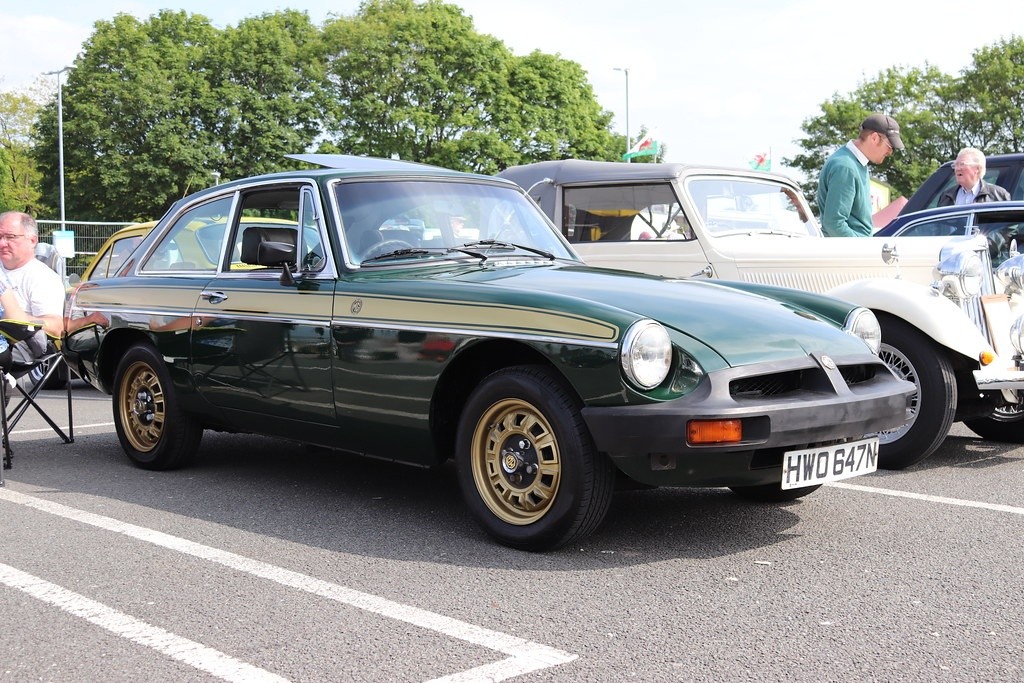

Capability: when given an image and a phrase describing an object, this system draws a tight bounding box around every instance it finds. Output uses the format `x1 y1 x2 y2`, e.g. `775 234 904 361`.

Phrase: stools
0 342 75 469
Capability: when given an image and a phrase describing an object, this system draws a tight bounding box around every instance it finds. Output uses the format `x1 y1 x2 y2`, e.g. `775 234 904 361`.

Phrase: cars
60 154 918 555
870 199 1024 272
476 161 1024 473
26 215 300 391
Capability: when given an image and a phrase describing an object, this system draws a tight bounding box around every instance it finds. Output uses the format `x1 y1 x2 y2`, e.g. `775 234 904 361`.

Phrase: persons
1 211 67 363
450 204 467 232
149 310 239 356
66 283 110 354
817 114 905 237
936 147 1020 268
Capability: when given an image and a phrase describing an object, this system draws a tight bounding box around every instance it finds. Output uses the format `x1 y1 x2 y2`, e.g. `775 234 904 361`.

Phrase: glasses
879 135 893 151
951 162 977 169
0 234 25 241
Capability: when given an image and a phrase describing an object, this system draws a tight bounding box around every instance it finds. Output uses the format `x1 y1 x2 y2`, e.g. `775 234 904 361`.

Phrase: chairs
241 227 297 270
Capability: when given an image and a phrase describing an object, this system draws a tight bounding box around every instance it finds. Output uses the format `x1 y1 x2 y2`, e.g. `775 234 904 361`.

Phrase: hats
862 115 904 149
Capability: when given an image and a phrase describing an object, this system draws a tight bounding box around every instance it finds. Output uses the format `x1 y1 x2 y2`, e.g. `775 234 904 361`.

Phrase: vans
898 152 1024 216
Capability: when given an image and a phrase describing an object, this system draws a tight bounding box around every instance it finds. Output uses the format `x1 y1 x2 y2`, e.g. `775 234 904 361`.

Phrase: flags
748 148 772 173
622 128 657 160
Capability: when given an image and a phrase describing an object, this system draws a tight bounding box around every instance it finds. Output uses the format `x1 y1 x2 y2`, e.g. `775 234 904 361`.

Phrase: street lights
613 66 630 164
40 64 79 284
